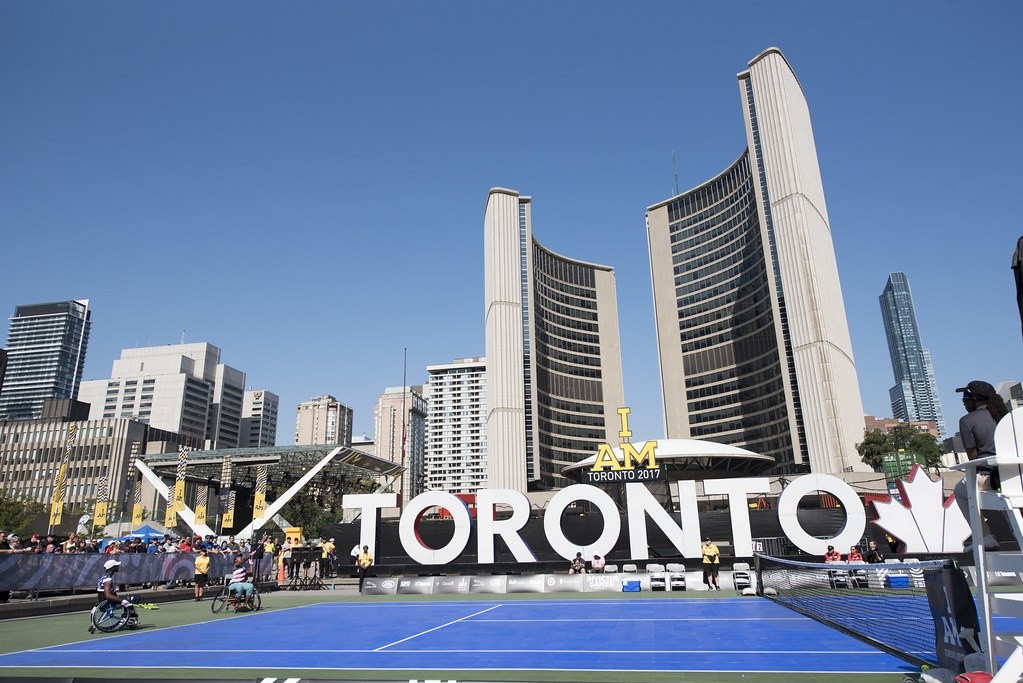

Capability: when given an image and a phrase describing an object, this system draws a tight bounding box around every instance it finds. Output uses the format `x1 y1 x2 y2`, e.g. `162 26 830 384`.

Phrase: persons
866 542 886 563
825 545 840 561
590 552 605 573
955 381 1010 551
97 560 138 618
358 545 373 593
0 532 336 598
194 548 212 601
350 544 360 565
227 550 257 608
568 552 586 574
848 546 863 560
701 537 721 591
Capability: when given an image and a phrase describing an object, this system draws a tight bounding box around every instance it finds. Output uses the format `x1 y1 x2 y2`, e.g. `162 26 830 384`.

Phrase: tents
112 525 176 549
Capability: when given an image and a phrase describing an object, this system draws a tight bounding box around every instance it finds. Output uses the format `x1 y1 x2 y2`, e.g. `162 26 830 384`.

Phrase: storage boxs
885 574 910 588
622 581 640 592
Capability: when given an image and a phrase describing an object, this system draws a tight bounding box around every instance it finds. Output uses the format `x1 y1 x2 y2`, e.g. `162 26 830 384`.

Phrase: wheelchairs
88 591 140 634
211 579 261 613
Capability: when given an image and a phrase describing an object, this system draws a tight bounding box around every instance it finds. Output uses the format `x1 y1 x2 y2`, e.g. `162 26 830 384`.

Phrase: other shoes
716 586 721 591
708 586 713 591
195 596 204 601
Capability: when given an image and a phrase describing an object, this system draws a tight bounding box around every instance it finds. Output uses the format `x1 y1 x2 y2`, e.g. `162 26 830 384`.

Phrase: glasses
961 397 973 402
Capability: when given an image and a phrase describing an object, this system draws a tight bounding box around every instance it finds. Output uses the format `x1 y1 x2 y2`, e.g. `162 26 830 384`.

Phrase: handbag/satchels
327 553 331 562
705 555 716 563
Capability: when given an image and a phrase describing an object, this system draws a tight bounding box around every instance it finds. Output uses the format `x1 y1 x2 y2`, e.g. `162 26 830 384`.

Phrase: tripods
284 561 329 591
249 559 271 594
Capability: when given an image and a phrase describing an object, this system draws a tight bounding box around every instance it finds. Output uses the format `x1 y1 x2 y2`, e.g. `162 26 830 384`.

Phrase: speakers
291 547 322 563
249 543 264 559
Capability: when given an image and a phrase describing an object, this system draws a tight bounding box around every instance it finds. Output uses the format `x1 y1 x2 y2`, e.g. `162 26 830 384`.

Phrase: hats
267 535 272 539
153 537 158 540
32 533 40 538
828 546 834 550
209 535 213 539
232 553 243 563
956 381 996 401
166 537 173 540
195 535 202 540
704 537 712 541
104 560 121 570
164 534 169 537
205 535 209 538
329 538 335 542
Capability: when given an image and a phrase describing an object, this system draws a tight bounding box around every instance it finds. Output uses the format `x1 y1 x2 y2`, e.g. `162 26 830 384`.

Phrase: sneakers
963 535 1000 553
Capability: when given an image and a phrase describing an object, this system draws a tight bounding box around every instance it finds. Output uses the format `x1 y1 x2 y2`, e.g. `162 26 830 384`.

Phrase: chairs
603 563 687 572
828 558 927 589
732 563 750 571
